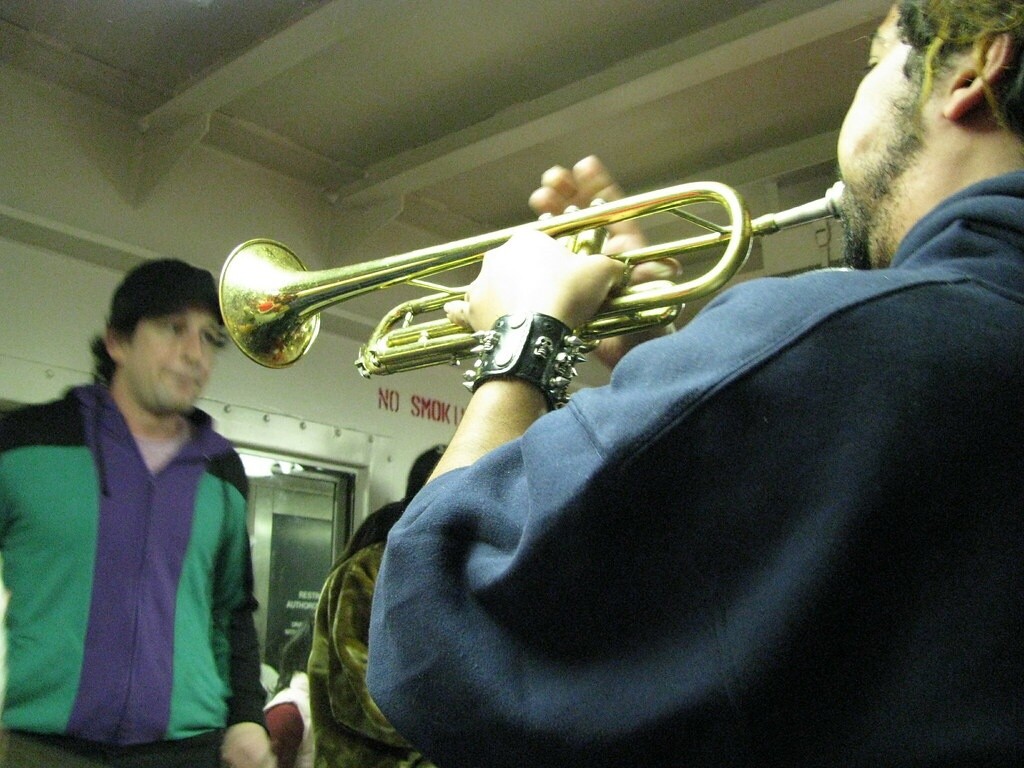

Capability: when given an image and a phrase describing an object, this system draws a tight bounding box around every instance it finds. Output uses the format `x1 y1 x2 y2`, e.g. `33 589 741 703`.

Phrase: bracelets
460 315 591 408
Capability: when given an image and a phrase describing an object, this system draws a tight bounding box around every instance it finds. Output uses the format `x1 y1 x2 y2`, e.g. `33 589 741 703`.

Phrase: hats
109 258 226 332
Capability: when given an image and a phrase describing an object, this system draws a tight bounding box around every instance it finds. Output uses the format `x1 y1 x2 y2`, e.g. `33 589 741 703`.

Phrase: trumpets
210 175 850 382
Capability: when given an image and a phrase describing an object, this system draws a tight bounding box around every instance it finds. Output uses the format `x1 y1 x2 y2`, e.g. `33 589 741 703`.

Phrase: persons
262 442 449 768
366 0 1024 768
0 259 276 768
